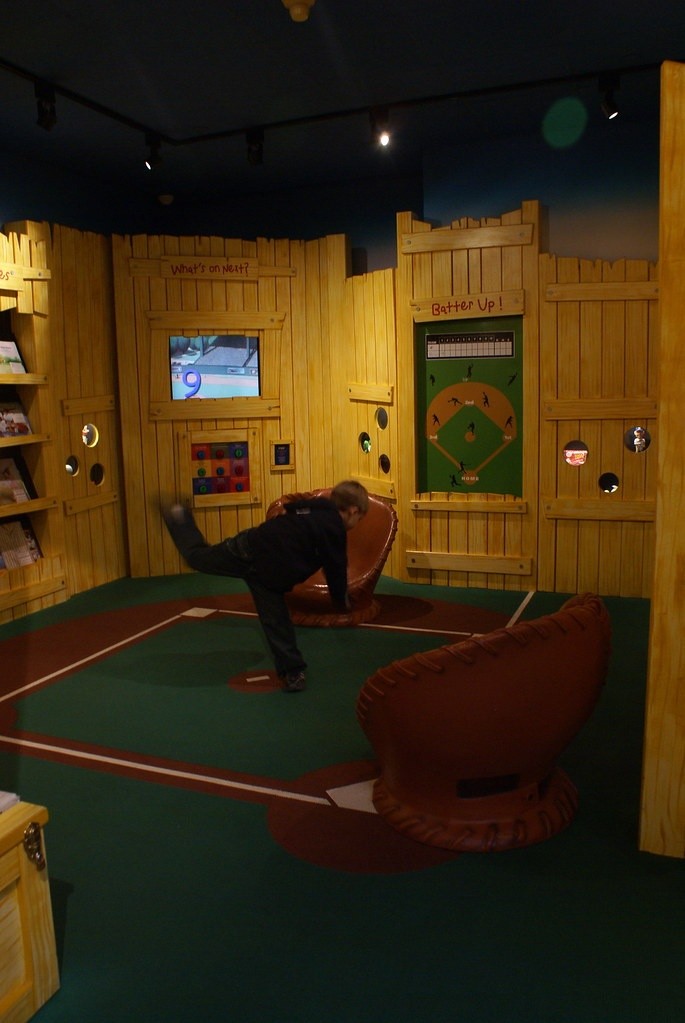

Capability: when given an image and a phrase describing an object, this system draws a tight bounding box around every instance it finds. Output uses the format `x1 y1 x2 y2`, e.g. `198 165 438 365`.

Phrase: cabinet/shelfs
0 231 66 627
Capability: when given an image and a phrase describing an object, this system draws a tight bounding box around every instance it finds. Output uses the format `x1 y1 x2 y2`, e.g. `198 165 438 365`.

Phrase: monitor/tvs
168 334 261 401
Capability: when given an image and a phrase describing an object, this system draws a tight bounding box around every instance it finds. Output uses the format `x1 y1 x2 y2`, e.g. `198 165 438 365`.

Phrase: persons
149 480 369 692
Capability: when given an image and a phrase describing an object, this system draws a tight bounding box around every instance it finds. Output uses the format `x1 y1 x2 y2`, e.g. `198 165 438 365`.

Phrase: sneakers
277 668 310 692
147 493 197 524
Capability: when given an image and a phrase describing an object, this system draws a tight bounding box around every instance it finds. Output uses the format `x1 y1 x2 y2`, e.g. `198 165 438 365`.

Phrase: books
1 330 44 571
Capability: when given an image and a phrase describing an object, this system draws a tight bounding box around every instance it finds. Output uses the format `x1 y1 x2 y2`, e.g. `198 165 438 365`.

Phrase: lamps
281 0 315 22
246 130 264 166
599 72 621 120
369 108 393 153
33 82 57 131
158 177 173 206
143 133 162 171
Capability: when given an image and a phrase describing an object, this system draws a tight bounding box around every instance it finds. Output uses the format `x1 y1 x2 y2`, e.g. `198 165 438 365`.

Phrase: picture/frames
176 427 262 512
269 439 295 471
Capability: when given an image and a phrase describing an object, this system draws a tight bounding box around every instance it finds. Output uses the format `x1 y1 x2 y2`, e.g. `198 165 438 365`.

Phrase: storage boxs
0 791 61 1023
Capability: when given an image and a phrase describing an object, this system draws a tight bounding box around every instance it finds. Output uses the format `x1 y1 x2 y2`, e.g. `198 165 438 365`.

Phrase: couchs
354 592 613 853
266 487 398 627
